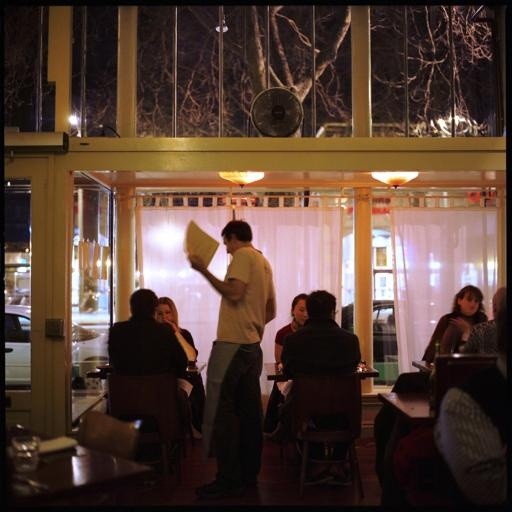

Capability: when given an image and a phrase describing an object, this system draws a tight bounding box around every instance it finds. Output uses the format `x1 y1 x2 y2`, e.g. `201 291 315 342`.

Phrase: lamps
369 171 419 188
218 171 265 189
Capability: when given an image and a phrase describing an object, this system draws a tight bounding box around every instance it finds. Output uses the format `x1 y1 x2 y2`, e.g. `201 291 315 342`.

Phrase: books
182 222 219 271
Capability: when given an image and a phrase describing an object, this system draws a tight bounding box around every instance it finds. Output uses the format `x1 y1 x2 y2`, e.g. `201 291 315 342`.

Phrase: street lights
68 106 87 138
214 6 229 138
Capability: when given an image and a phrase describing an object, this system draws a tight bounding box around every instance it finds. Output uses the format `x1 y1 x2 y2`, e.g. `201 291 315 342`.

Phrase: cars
6 305 110 390
342 300 400 384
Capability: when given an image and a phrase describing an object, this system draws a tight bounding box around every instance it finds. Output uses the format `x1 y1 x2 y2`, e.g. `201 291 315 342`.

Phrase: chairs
0 358 512 512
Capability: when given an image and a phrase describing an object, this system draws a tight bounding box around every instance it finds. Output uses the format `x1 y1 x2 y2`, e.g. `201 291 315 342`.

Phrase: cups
11 435 39 478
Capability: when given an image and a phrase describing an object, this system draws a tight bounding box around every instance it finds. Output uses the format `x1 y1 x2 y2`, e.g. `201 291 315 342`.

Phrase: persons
155 296 206 445
449 287 507 352
187 221 277 494
278 290 362 484
274 294 308 364
108 289 189 468
431 307 508 505
421 286 488 364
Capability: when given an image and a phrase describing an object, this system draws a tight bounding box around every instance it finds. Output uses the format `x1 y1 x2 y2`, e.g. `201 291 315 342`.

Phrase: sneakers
305 470 334 485
196 481 234 496
